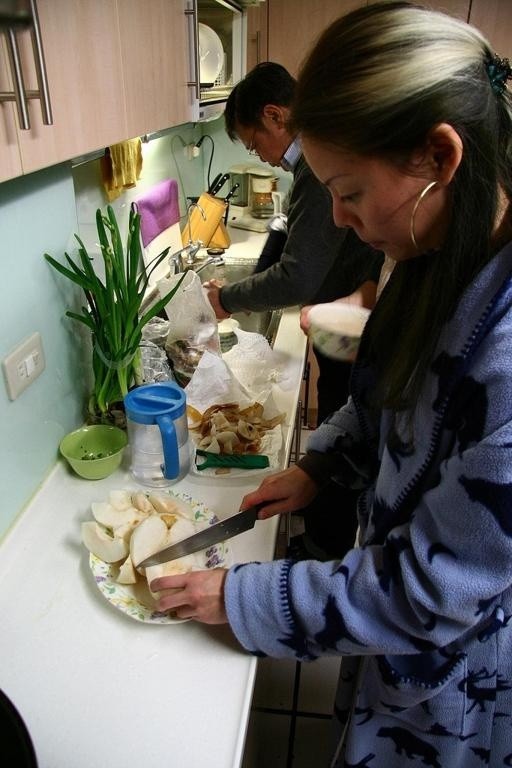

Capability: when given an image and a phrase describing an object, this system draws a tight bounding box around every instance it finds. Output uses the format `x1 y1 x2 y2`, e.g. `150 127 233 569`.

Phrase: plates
86 486 240 626
198 23 225 84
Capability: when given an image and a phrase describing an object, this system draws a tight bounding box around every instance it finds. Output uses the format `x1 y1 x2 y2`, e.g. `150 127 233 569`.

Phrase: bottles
247 166 280 219
225 162 253 207
123 381 192 490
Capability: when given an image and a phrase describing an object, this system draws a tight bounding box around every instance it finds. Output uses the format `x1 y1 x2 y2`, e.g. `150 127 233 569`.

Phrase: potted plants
39 201 189 431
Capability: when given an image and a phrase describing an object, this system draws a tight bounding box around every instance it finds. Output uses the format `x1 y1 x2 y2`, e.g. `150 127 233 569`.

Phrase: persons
300 251 397 345
149 0 512 768
196 61 386 430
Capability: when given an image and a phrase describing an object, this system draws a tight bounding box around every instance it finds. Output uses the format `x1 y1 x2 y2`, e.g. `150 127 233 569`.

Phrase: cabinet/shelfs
114 0 249 145
1 0 126 185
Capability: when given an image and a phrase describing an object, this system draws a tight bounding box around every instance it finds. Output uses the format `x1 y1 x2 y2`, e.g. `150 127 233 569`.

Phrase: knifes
207 172 241 205
132 497 289 570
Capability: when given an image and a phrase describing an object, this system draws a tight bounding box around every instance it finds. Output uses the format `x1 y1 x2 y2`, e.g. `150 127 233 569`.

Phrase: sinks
180 256 285 348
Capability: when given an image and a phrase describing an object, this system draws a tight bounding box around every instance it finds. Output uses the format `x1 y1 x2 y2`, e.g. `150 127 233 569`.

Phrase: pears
80 487 207 603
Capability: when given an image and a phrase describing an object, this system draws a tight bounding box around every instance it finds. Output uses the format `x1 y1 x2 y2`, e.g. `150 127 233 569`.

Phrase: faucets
187 203 206 245
167 244 215 276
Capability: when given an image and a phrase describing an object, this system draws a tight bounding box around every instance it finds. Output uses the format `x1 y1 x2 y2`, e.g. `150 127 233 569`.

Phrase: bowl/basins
306 302 373 361
59 423 129 481
